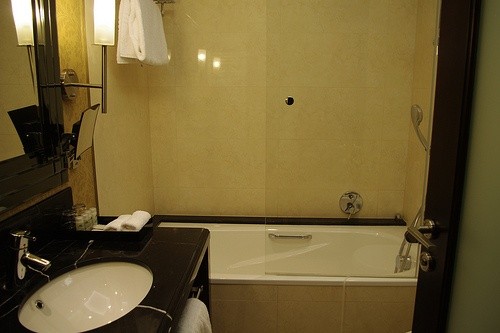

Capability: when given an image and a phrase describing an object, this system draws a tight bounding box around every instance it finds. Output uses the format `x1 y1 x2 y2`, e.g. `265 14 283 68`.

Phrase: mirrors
0 0 69 215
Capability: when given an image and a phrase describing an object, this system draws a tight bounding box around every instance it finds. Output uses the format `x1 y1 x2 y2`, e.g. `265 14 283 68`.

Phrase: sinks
18 256 154 333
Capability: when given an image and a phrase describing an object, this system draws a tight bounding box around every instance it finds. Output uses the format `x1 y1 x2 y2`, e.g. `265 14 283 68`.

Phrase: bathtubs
158 222 421 286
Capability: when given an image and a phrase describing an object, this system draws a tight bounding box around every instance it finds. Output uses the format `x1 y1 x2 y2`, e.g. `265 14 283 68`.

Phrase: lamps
60 0 116 113
10 0 37 96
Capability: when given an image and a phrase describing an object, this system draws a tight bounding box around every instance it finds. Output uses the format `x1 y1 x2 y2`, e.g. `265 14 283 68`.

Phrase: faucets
410 104 431 152
10 228 52 281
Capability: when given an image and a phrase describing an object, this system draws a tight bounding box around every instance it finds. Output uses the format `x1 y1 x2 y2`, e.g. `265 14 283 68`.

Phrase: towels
177 297 212 333
120 210 151 232
103 214 132 231
116 0 169 66
